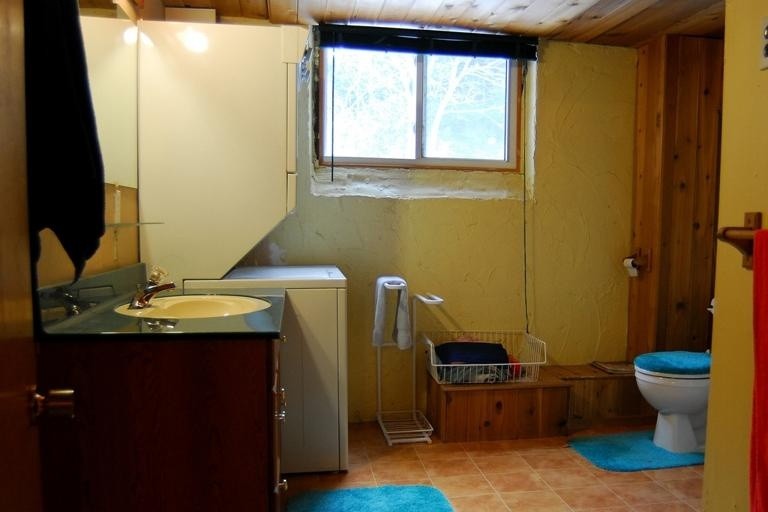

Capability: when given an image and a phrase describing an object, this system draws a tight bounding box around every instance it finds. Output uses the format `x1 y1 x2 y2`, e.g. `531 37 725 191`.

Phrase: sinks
115 294 272 321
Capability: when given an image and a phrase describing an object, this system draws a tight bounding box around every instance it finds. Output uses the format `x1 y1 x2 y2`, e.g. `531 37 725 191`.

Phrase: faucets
127 266 175 308
145 320 177 331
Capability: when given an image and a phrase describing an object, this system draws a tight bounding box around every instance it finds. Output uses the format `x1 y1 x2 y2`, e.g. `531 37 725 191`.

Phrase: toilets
633 299 715 452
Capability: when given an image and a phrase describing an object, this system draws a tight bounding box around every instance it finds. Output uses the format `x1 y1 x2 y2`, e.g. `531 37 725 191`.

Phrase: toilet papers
623 257 639 277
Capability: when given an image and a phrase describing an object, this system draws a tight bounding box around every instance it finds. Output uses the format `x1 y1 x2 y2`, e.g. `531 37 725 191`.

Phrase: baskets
420 328 547 384
376 409 433 438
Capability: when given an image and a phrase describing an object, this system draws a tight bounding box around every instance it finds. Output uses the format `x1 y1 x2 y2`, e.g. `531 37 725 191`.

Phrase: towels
372 277 412 350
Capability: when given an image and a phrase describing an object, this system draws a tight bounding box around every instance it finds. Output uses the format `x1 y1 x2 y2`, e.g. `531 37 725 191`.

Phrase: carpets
566 429 704 472
284 485 455 512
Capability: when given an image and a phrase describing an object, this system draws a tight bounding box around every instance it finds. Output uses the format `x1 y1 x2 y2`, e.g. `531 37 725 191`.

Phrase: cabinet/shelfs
38 338 289 512
137 19 299 294
181 266 347 473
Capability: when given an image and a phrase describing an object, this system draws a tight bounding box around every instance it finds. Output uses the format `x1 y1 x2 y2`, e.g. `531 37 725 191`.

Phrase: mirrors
32 0 140 290
